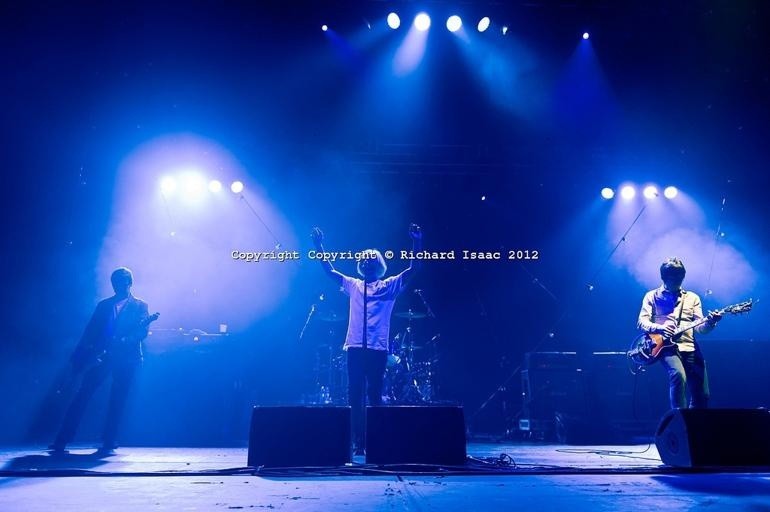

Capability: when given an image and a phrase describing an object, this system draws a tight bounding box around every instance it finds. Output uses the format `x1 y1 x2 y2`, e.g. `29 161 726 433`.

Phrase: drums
385 354 405 395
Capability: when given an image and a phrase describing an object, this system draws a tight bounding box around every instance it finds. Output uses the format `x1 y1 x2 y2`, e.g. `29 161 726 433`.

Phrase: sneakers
98 442 117 455
48 444 66 454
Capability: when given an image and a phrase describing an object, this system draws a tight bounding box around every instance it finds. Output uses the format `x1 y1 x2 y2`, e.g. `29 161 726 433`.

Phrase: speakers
654 407 770 468
247 405 354 468
364 405 467 465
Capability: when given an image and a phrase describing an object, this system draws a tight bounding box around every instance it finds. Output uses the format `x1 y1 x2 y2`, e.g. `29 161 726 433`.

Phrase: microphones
363 260 368 268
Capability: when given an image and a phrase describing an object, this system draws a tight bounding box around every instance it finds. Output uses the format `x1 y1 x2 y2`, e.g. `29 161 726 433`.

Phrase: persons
309 222 423 456
636 257 722 409
47 267 150 458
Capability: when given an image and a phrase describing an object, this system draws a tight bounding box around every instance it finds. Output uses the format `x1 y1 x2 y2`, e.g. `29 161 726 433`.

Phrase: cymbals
318 314 347 323
393 310 427 319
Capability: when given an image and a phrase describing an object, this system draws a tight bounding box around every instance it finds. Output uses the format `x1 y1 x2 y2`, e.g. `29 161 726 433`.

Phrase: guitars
627 299 752 365
79 311 159 373
